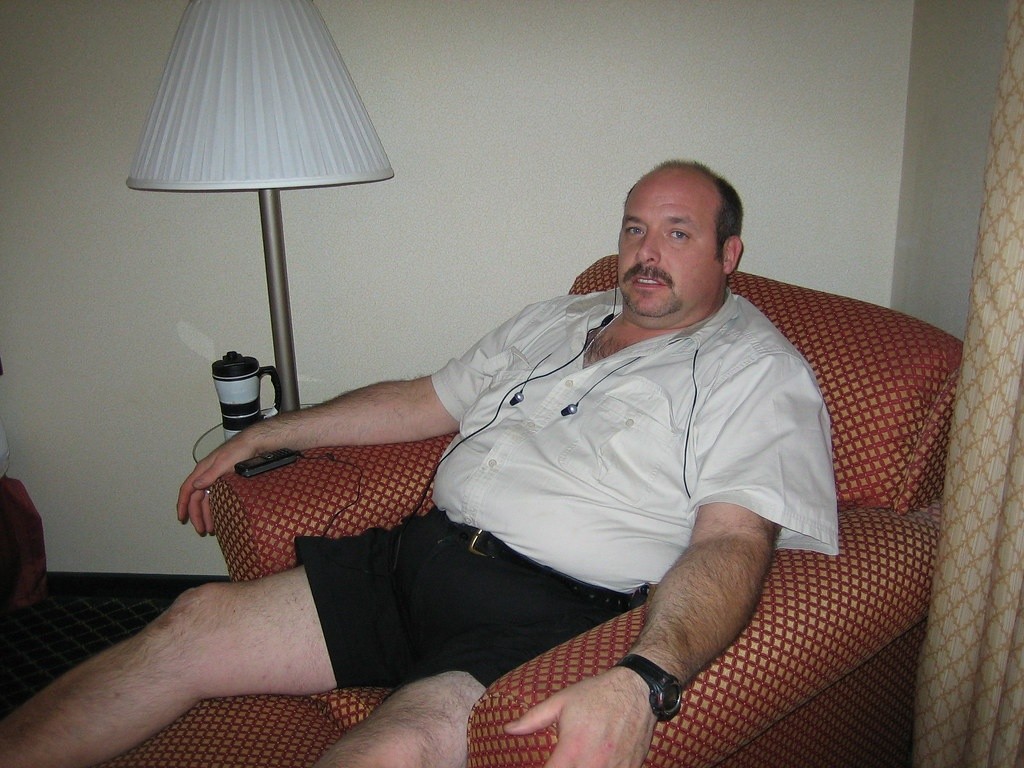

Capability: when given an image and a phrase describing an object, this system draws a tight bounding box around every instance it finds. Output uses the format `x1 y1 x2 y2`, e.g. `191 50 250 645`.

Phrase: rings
205 489 210 494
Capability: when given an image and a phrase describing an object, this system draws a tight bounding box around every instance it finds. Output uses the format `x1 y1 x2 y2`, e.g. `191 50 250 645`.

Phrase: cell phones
234 448 299 477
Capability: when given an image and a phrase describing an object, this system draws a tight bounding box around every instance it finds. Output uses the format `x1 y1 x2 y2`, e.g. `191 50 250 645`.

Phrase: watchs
612 654 682 722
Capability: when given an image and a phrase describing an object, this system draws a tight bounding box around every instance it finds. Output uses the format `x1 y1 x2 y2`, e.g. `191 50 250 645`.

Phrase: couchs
100 252 964 768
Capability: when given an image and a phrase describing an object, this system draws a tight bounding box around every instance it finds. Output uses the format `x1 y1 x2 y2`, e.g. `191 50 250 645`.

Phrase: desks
192 403 319 463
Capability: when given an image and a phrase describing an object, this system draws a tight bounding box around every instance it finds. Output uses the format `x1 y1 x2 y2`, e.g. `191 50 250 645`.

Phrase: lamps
126 0 396 412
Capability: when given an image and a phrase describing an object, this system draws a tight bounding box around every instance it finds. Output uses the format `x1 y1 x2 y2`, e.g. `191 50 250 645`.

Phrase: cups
212 351 282 437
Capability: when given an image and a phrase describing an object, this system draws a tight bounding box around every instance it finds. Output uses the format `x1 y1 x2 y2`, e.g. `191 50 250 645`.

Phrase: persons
0 159 839 768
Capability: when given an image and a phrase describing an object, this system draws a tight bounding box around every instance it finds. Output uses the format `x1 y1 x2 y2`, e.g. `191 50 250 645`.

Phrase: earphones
509 392 524 406
562 404 577 416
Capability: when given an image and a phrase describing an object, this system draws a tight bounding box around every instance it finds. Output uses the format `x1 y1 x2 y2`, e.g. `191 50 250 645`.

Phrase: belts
425 505 631 612
